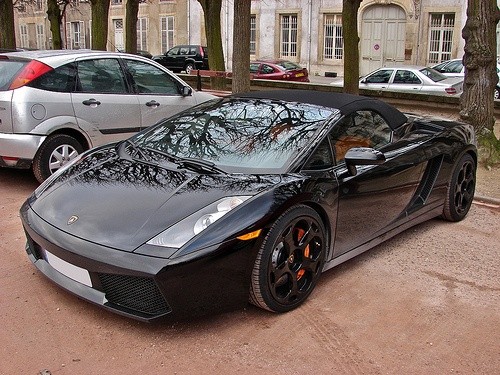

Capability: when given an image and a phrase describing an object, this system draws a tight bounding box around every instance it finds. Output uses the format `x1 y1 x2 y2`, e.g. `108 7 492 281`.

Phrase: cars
431 55 500 99
18 47 35 54
329 67 463 98
226 57 310 83
118 50 153 60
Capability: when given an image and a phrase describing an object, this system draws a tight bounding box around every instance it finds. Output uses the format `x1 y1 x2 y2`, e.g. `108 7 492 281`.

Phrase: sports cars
18 88 478 322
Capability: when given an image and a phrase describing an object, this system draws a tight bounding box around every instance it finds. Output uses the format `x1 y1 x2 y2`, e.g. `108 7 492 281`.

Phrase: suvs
151 45 207 75
0 50 222 181
